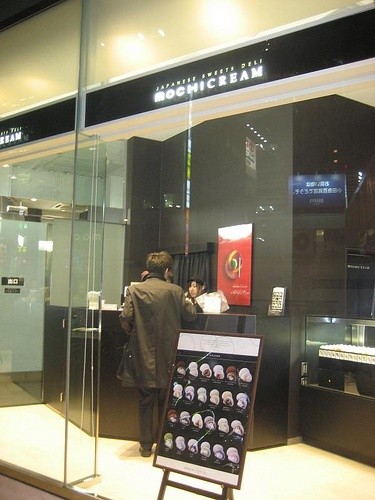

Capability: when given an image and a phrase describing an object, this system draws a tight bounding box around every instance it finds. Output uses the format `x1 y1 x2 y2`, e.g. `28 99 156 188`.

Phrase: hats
186 274 205 285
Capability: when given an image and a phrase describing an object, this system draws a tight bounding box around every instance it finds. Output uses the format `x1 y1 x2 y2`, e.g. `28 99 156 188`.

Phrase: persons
117 251 196 460
165 268 207 313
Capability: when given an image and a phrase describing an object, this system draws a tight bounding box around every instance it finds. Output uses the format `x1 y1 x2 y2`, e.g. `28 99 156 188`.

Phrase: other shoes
139 446 151 457
